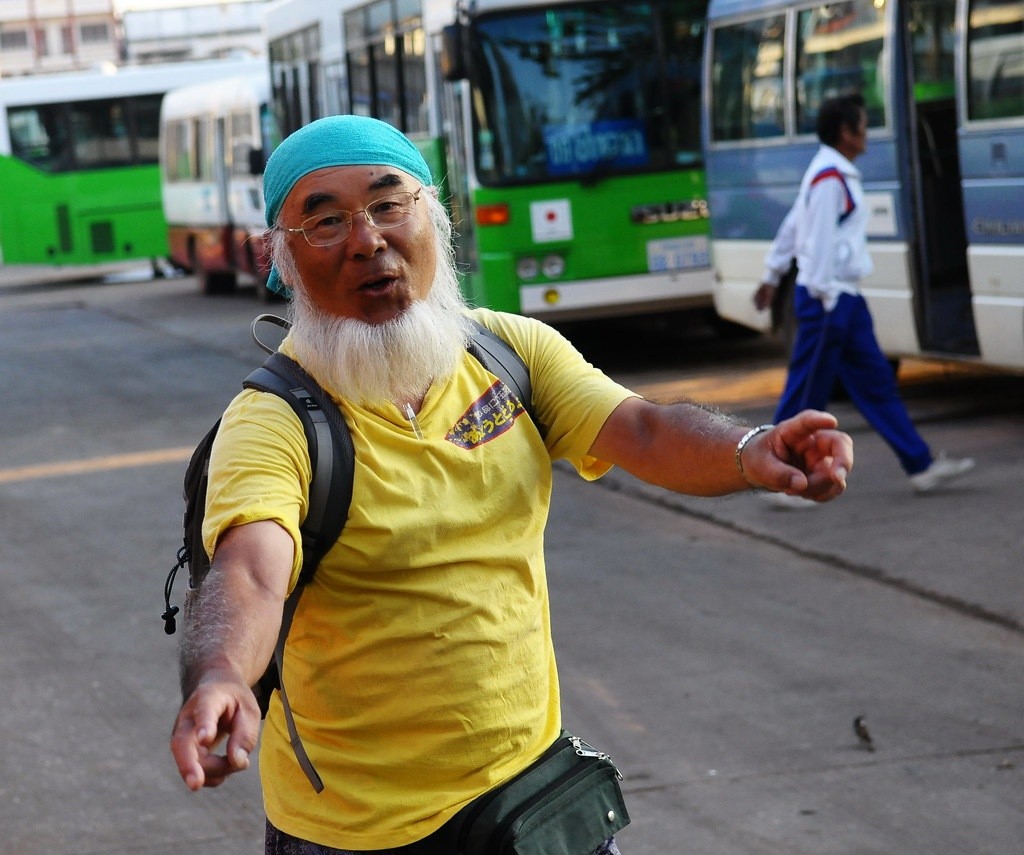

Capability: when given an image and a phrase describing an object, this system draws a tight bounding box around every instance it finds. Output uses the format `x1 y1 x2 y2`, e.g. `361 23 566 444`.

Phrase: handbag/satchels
441 725 632 855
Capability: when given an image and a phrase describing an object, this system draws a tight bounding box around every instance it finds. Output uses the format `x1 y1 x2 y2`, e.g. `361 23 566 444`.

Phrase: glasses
276 185 423 248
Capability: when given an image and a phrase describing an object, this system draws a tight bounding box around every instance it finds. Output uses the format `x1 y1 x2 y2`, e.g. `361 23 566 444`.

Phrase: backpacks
183 308 546 724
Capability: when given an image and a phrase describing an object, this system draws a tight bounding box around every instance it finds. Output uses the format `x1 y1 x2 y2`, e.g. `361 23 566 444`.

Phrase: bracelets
736 423 775 489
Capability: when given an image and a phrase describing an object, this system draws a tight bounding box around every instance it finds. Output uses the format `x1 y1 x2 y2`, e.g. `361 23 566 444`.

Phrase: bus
158 75 280 301
0 51 264 284
699 0 1024 400
262 1 712 327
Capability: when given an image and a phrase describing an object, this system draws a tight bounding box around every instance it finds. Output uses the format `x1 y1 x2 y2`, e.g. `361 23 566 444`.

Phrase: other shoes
911 451 975 492
760 489 818 511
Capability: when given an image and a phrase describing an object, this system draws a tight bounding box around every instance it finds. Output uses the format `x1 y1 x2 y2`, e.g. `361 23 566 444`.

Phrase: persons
838 49 860 75
754 92 978 510
168 114 853 855
